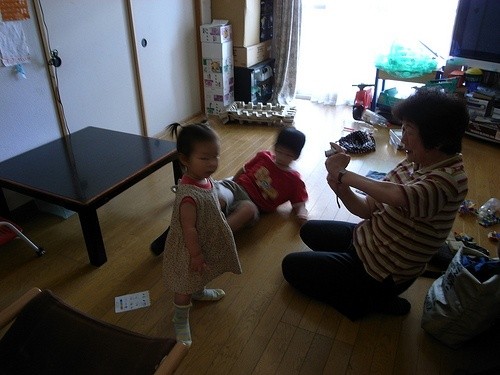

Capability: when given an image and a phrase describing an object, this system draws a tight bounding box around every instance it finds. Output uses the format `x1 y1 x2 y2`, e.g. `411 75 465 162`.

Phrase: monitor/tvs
447 0 500 74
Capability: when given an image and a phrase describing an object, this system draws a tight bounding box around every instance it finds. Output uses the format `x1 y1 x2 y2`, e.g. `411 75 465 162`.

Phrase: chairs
0 287 188 375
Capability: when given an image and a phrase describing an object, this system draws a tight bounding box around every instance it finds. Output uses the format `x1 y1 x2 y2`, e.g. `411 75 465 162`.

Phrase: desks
371 65 441 111
0 126 184 267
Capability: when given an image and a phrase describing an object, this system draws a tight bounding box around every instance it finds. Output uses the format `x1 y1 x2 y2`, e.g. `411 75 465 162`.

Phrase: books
473 90 494 101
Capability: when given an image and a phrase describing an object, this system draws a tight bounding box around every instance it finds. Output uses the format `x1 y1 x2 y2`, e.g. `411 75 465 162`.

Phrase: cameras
325 146 347 157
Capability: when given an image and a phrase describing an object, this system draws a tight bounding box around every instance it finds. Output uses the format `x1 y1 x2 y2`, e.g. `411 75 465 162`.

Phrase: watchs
337 169 348 184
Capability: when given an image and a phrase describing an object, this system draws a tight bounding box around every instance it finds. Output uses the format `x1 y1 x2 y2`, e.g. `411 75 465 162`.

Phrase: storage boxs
200 24 233 116
211 0 272 68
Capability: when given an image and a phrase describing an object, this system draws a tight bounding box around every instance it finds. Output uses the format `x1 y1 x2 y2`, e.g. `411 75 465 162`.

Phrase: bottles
478 198 500 218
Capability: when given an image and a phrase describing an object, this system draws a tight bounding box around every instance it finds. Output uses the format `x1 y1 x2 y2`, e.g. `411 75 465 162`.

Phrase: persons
160 113 242 350
149 125 309 257
282 85 468 322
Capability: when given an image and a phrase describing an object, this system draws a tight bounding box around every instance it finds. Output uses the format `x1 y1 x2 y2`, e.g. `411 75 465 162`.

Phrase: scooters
352 83 376 121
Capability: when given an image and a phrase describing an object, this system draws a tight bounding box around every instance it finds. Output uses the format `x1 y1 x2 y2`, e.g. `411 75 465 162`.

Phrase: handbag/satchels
422 246 500 350
